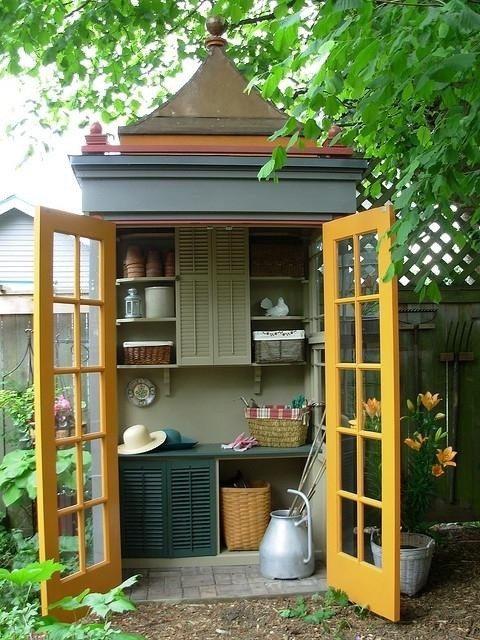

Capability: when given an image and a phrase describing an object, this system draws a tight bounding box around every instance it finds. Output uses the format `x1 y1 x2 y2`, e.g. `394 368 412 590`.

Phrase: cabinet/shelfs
114 228 178 369
246 226 315 368
119 454 221 560
175 225 251 367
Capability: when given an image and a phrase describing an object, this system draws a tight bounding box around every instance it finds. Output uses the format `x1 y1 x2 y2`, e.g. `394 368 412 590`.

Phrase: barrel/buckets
143 287 175 318
258 489 316 579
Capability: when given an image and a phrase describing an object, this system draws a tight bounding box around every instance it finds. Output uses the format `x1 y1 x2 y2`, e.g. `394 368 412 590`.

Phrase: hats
156 429 198 450
118 424 168 457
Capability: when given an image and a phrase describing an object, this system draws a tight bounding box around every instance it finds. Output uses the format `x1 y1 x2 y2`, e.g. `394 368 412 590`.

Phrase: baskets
244 404 312 448
253 330 307 364
219 478 272 551
122 340 174 365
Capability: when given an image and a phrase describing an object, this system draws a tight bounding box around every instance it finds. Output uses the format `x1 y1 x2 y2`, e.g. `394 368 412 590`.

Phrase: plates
126 377 156 407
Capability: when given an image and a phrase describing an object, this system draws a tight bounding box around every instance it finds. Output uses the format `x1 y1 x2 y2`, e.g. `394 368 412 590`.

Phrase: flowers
349 386 459 548
55 394 73 429
